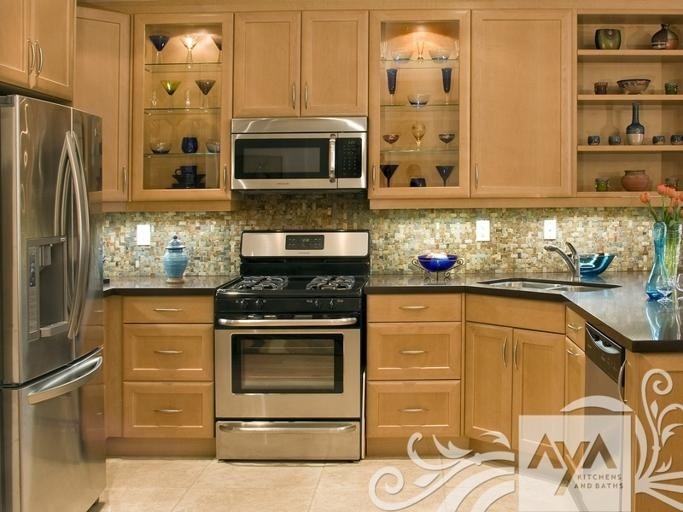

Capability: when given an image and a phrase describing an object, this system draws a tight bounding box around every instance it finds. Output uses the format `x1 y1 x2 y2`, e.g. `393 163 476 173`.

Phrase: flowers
639 182 682 246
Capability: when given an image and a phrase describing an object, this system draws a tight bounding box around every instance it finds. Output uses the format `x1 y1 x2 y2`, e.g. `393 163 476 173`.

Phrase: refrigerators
0 92 104 512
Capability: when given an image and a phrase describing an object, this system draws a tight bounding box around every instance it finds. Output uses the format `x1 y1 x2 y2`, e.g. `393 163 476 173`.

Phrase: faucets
543 245 575 273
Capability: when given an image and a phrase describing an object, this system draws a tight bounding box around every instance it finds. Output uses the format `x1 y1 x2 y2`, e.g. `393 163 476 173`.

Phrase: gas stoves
211 273 367 313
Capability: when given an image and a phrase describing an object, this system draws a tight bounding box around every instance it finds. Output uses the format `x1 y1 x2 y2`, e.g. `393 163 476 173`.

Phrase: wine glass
653 272 683 304
210 33 223 63
654 305 683 331
380 164 398 187
435 164 455 187
159 78 181 109
438 132 456 150
386 67 398 106
453 39 461 59
440 67 453 104
406 122 427 152
381 39 389 60
415 37 426 61
178 34 202 64
382 134 400 150
148 33 170 64
194 78 217 108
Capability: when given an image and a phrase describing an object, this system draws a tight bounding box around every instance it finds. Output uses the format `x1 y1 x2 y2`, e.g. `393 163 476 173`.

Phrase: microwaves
229 132 368 192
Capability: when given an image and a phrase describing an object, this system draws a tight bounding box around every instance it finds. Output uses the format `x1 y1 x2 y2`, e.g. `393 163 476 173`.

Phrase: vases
643 222 669 300
660 222 679 304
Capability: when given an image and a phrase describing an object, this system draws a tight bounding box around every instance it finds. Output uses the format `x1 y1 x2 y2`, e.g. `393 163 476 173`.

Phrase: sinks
491 280 560 291
548 284 602 294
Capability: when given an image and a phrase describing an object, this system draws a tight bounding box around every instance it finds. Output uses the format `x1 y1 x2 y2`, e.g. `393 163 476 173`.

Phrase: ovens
212 315 364 421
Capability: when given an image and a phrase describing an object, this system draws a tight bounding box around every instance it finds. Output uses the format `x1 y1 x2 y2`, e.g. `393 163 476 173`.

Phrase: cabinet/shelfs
462 1 576 208
564 0 682 210
123 0 247 212
561 305 586 494
367 294 467 465
233 1 367 119
103 294 215 459
465 292 566 480
357 1 468 210
62 6 132 216
617 348 683 512
0 1 78 104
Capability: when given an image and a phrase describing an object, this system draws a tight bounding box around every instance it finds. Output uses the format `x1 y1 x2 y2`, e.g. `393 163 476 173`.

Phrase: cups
595 176 609 192
173 164 199 174
666 176 681 191
593 80 609 95
180 136 198 152
409 177 427 187
205 136 221 153
670 134 683 145
664 81 678 95
586 135 600 145
148 133 172 154
652 134 666 145
609 135 622 145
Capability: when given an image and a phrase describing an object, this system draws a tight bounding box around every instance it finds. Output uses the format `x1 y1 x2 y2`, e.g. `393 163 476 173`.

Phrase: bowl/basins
617 79 651 94
172 172 206 183
417 254 458 273
427 48 452 63
390 49 414 63
406 92 432 106
571 253 616 276
